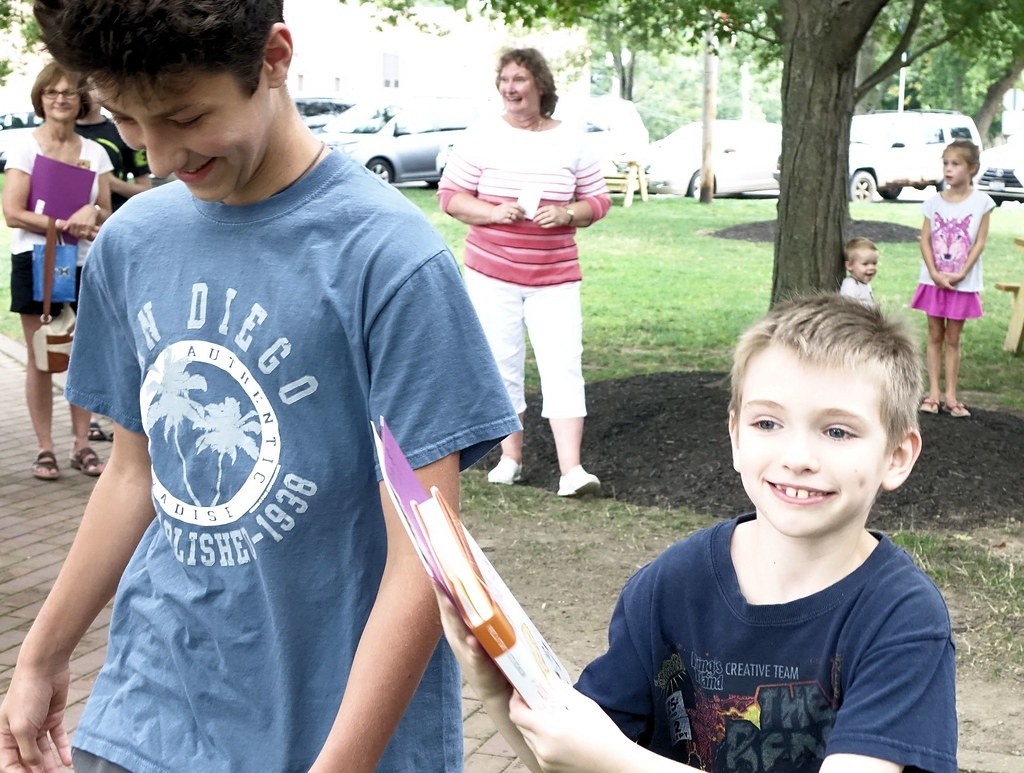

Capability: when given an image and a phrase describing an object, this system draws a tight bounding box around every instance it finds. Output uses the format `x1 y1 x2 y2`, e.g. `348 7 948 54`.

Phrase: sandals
85 421 107 441
69 447 106 476
31 451 61 479
919 398 941 414
941 400 972 418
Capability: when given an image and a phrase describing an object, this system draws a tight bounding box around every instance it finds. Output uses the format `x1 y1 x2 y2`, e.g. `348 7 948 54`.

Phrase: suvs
850 109 983 200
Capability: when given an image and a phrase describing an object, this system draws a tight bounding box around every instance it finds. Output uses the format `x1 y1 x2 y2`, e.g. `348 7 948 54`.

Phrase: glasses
38 88 80 100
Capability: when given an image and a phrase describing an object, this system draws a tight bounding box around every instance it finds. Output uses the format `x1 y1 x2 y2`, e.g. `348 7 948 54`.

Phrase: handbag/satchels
31 304 76 373
31 219 79 303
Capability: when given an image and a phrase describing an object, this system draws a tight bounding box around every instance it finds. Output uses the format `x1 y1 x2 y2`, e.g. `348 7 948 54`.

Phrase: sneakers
556 465 602 501
486 455 523 485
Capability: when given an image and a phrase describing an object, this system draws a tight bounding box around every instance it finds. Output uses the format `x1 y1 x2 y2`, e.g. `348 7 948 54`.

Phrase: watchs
562 204 575 228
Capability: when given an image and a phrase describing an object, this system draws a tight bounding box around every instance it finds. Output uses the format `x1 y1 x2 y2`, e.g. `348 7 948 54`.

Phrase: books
370 416 573 711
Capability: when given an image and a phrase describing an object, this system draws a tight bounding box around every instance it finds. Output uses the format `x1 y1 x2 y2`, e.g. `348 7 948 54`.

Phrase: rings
508 213 512 219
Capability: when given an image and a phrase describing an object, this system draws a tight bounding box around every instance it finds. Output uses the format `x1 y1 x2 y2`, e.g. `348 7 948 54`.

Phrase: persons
843 235 878 309
434 285 957 772
0 0 523 773
909 139 997 418
436 47 613 496
0 62 154 481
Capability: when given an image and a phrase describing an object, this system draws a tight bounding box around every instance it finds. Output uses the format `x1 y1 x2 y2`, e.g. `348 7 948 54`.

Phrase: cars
317 86 500 186
560 96 646 168
646 116 783 201
973 132 1024 208
0 108 44 169
294 97 356 132
